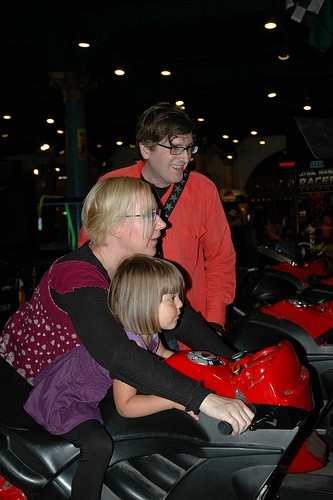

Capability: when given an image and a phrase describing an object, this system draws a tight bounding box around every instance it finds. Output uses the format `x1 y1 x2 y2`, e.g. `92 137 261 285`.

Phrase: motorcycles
230 228 333 299
222 298 333 376
0 339 332 500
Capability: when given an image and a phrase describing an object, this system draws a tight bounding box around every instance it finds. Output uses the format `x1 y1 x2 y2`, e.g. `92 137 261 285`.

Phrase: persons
77 104 235 351
302 216 333 258
0 176 255 440
23 256 200 500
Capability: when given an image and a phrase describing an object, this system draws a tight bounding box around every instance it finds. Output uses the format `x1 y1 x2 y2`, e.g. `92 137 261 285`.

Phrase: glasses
157 143 199 155
125 209 162 222
